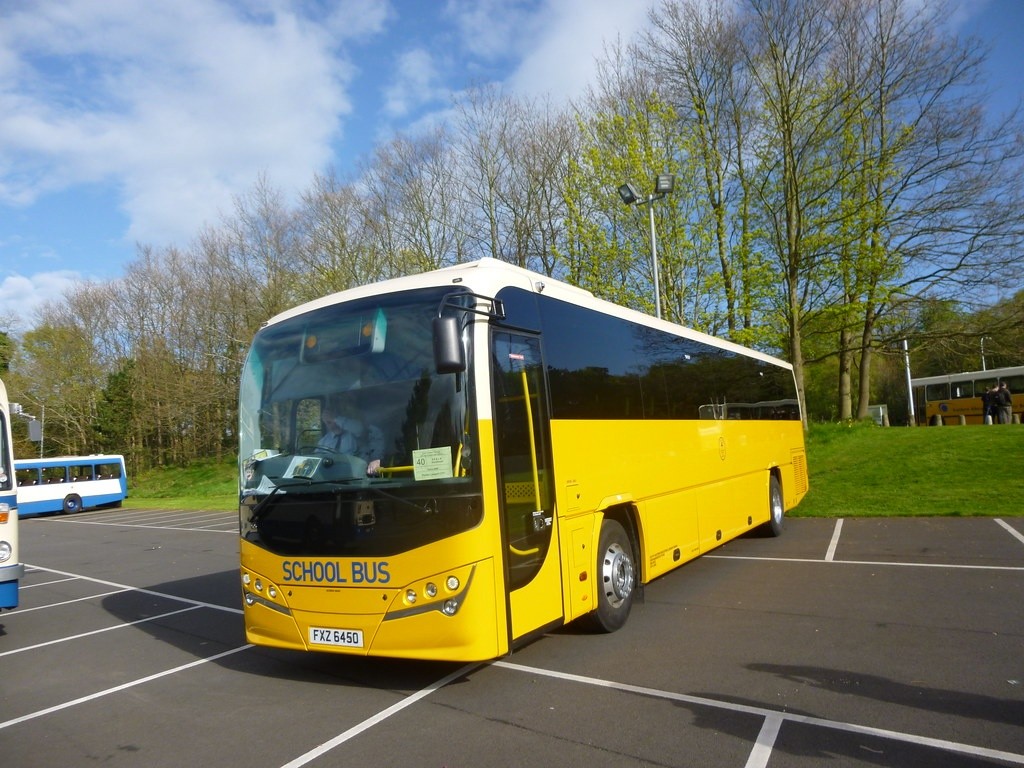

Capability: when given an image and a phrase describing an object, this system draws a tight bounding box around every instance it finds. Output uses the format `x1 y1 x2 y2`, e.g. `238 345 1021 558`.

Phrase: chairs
16 473 114 487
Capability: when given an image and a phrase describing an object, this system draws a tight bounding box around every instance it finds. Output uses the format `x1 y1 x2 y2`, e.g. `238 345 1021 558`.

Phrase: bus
906 366 1024 425
236 258 811 667
14 454 128 519
0 381 44 614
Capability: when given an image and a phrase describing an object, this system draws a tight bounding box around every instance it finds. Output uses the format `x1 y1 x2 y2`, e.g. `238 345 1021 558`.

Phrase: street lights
980 337 993 371
619 174 674 319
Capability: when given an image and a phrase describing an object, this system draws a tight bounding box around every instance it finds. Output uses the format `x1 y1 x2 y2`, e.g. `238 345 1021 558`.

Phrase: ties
334 436 341 451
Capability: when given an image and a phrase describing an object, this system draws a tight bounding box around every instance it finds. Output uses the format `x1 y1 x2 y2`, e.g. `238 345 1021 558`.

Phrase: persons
981 382 1012 424
309 402 386 474
